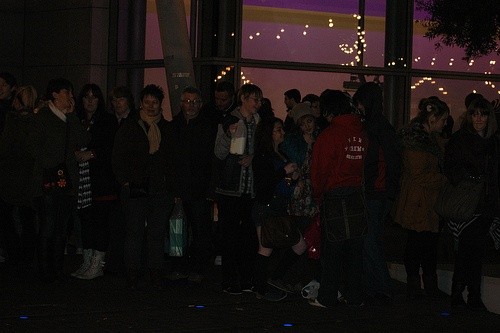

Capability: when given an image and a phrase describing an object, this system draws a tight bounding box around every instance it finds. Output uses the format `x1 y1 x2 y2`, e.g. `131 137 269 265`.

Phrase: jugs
228 119 248 155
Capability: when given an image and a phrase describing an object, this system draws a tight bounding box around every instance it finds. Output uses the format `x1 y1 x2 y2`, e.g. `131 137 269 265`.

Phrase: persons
308 89 367 308
433 99 500 312
351 82 401 303
102 87 139 272
69 83 118 280
389 100 450 300
121 84 179 288
242 117 298 301
163 87 211 284
0 69 47 266
30 78 79 291
199 80 360 146
1 84 57 279
214 84 266 298
419 92 500 144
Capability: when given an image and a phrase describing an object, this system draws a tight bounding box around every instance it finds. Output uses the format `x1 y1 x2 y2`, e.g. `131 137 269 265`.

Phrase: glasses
246 95 265 104
181 98 201 104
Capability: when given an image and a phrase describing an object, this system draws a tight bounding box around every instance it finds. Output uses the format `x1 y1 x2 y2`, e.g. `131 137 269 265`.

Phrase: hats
292 103 313 127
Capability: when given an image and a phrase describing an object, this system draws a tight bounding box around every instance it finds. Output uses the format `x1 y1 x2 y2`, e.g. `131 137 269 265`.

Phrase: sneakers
248 277 296 301
307 298 327 308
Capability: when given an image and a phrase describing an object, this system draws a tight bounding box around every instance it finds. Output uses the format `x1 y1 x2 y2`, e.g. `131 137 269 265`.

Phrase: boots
70 249 105 280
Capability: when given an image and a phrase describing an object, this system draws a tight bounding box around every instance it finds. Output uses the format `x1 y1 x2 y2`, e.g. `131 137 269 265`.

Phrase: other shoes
407 289 486 311
340 296 394 325
